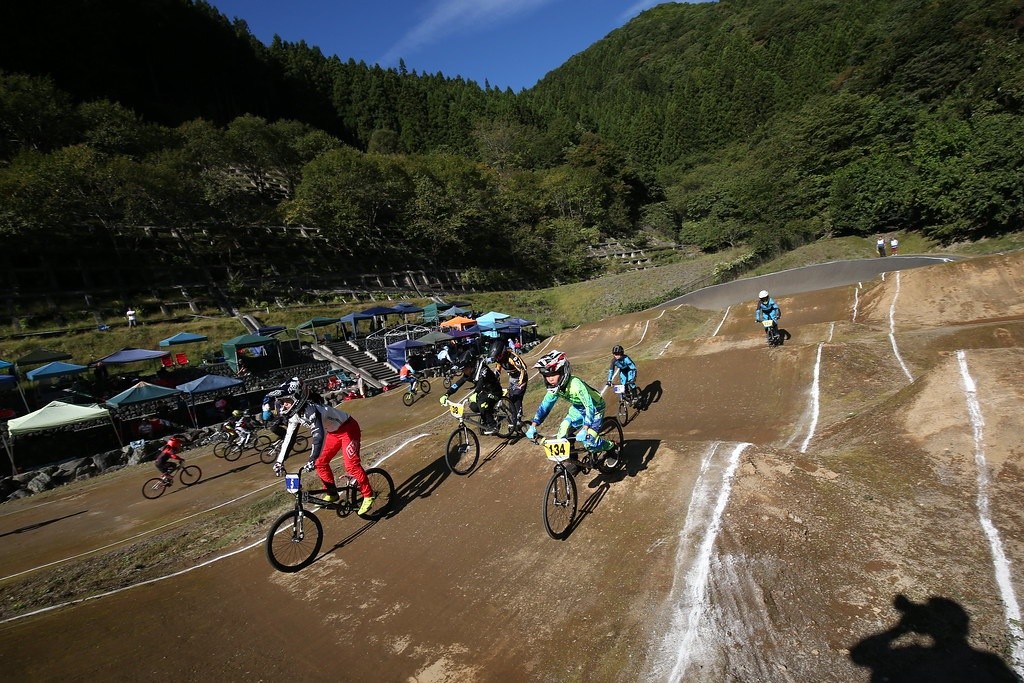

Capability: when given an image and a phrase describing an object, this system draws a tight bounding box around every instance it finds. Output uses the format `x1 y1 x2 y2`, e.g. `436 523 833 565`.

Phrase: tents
8 400 122 475
92 346 175 379
420 300 476 331
387 312 537 372
160 332 208 351
296 317 347 349
0 359 30 414
16 350 94 405
341 303 424 340
221 334 282 375
252 325 293 355
105 381 195 443
176 374 250 426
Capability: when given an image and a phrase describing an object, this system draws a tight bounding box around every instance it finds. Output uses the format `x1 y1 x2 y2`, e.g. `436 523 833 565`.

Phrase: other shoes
483 421 498 435
358 492 377 515
562 458 581 467
631 397 638 405
314 494 339 508
163 473 174 480
607 440 619 468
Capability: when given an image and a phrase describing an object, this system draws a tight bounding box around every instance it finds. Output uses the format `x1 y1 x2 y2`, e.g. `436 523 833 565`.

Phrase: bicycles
264 462 396 574
755 317 781 348
403 372 431 407
141 459 202 500
529 415 624 540
210 419 308 464
605 380 644 427
440 390 518 476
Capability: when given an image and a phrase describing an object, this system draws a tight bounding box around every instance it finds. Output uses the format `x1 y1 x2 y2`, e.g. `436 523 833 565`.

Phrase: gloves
273 462 286 477
575 429 588 442
526 426 538 439
303 460 315 472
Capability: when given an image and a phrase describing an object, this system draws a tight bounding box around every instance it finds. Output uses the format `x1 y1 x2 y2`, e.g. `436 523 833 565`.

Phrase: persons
94 362 108 392
877 236 887 257
377 318 384 330
756 290 781 342
138 400 174 438
468 309 483 319
399 338 637 478
127 307 136 328
236 359 250 375
356 373 364 398
213 396 226 419
68 377 92 398
890 237 898 256
154 377 376 516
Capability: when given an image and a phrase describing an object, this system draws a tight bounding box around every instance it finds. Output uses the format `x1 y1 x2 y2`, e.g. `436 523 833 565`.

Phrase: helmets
244 409 254 417
449 350 477 377
758 290 769 299
532 350 571 395
489 340 507 363
612 346 624 361
232 410 242 417
166 438 181 452
265 375 310 420
